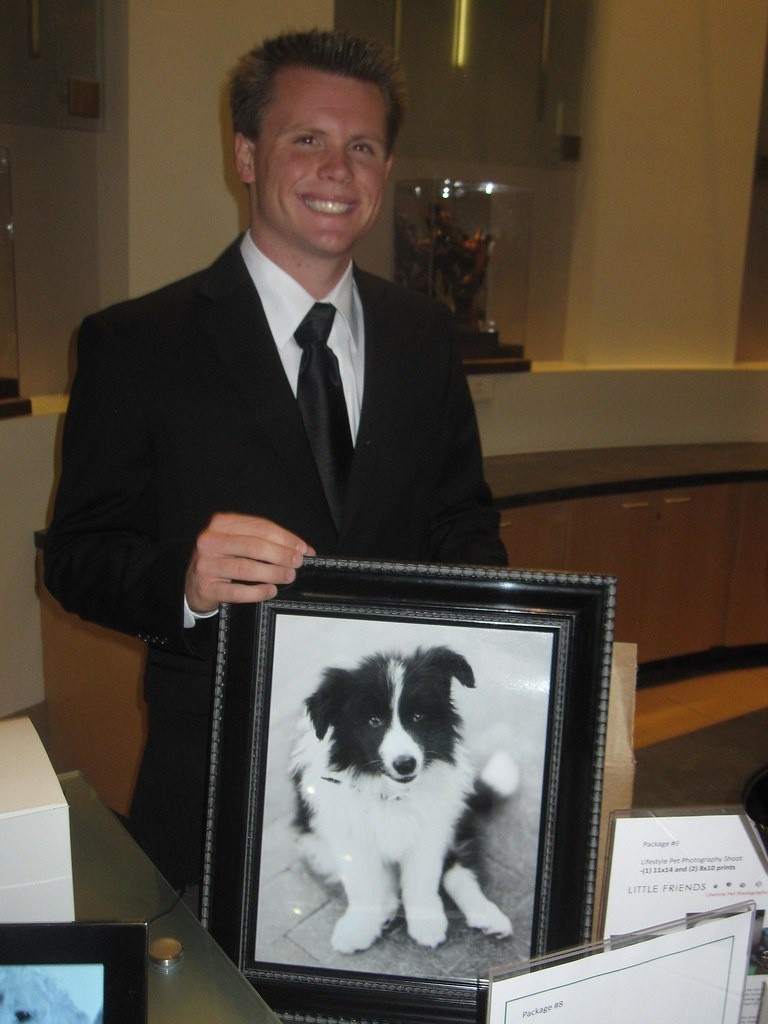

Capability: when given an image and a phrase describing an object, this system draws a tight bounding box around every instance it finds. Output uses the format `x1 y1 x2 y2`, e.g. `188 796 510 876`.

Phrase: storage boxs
0 716 76 925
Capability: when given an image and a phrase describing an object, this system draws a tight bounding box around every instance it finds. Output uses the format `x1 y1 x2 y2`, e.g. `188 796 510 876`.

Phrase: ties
294 302 354 532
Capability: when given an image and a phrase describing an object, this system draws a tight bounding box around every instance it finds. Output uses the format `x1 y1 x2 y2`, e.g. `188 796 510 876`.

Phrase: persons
393 197 493 327
45 27 509 919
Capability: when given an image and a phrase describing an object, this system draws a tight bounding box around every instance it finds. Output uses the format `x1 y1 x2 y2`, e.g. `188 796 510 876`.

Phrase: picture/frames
0 921 150 1024
198 553 617 1023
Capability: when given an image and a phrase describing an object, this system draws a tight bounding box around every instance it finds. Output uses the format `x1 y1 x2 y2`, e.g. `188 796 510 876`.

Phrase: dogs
287 647 525 959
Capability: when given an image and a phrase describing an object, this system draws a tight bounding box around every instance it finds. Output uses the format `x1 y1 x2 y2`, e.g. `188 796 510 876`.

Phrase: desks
58 771 284 1024
